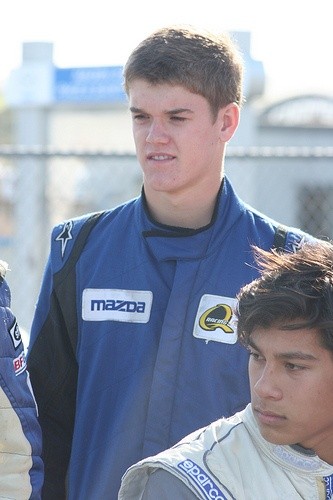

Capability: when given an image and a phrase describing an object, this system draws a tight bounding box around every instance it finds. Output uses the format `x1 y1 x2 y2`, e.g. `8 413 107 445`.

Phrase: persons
0 261 48 500
115 234 333 500
21 23 316 500
